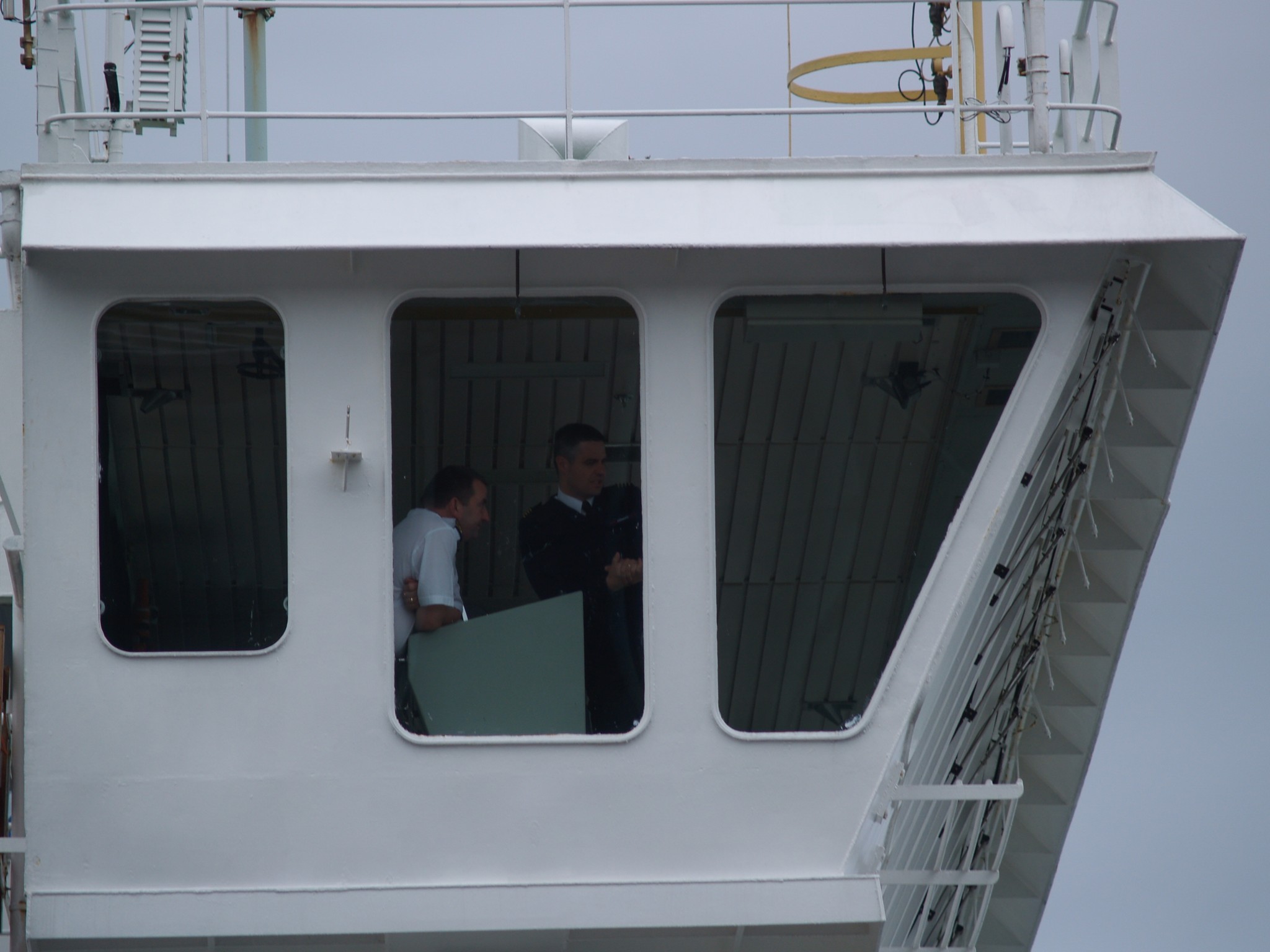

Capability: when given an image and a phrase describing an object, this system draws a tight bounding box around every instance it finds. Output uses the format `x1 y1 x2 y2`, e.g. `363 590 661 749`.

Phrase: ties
581 501 592 517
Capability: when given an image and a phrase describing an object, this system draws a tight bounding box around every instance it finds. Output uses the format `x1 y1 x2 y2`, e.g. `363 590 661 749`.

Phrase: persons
518 423 644 736
393 464 492 734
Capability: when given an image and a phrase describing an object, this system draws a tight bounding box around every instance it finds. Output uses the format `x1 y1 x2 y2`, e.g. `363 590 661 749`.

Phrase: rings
410 597 413 604
